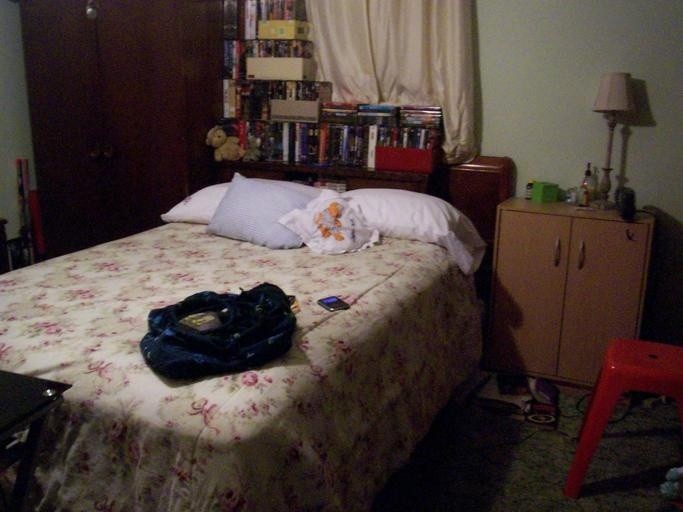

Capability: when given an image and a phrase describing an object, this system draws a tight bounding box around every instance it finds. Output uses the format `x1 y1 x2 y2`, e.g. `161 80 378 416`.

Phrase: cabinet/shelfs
19 0 225 259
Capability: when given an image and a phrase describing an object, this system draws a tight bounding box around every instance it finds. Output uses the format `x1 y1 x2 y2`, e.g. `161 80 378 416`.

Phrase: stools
563 339 683 500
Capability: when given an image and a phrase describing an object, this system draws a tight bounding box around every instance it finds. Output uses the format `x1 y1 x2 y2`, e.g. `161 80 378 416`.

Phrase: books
357 105 398 127
223 40 246 80
282 122 378 172
319 102 370 126
246 40 314 58
259 0 306 22
223 80 273 120
272 81 331 101
239 121 282 161
399 105 442 130
223 0 259 40
378 128 440 151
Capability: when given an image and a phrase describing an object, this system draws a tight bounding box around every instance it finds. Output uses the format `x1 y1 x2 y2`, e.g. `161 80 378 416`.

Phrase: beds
0 156 519 512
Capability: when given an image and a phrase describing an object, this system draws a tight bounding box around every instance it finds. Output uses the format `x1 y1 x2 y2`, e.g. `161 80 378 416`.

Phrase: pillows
160 182 227 224
277 187 378 256
208 173 321 249
341 187 487 276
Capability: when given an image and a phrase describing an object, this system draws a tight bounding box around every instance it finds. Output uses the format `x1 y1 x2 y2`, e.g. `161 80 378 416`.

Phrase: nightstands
486 195 656 389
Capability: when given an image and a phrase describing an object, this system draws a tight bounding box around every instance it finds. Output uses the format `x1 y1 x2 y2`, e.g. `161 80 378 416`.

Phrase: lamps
589 73 636 211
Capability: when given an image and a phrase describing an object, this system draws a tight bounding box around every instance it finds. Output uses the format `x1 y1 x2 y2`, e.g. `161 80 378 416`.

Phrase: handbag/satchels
139 281 297 383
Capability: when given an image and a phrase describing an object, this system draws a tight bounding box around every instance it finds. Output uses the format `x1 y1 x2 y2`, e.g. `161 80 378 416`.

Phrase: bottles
525 178 534 200
580 162 596 200
579 185 590 206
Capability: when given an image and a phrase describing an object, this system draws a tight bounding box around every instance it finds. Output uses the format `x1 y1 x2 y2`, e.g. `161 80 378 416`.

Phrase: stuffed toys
205 126 246 161
242 133 282 163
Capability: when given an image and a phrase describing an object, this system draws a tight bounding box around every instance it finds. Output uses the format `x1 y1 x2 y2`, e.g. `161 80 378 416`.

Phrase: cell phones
317 295 350 312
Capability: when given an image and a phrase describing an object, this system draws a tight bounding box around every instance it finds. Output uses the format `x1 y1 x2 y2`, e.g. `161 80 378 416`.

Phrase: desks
0 368 73 512
